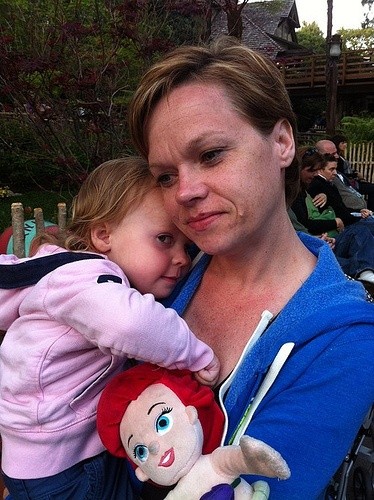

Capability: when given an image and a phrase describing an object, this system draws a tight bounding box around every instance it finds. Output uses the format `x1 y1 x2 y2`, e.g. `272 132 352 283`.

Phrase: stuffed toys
96 359 292 500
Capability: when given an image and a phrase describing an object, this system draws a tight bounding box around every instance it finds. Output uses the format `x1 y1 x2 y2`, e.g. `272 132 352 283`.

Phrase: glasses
303 147 318 160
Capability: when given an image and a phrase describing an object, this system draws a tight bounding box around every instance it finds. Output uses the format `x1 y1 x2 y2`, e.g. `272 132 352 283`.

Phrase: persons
289 134 374 298
125 33 374 500
0 155 222 500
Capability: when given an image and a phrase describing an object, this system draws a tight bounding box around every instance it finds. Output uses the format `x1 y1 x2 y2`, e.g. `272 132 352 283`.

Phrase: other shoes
358 270 374 284
